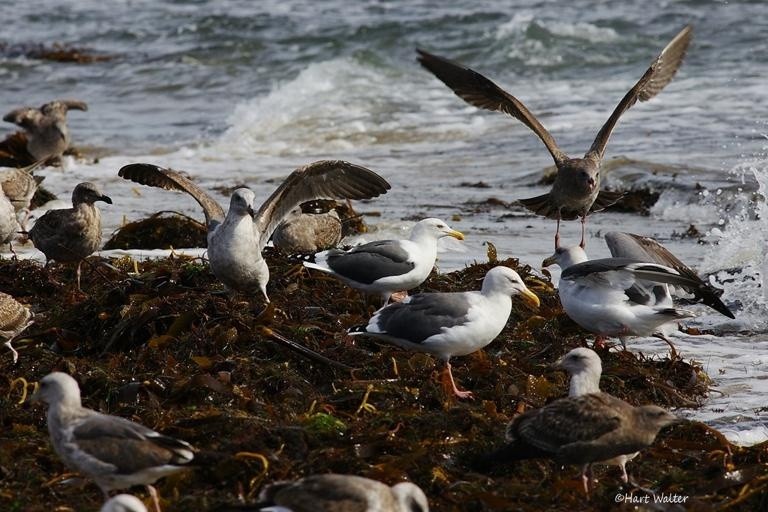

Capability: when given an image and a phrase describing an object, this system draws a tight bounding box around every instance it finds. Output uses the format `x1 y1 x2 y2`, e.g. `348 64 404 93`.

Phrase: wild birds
259 472 429 512
325 218 466 310
500 348 679 500
347 265 541 403
30 372 200 512
117 160 391 306
0 98 114 299
0 291 35 368
99 492 148 512
413 25 694 254
539 231 735 359
